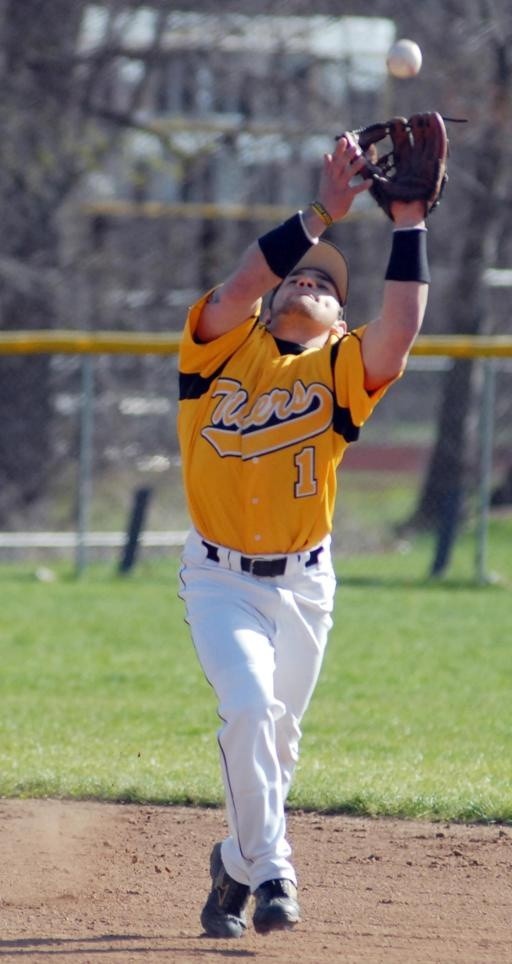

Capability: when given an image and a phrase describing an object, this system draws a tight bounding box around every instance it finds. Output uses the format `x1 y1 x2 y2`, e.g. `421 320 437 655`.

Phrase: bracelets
256 208 320 283
382 224 432 285
308 198 336 230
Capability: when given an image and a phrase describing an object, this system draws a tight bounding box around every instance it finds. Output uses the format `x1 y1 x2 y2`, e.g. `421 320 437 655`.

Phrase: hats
288 236 350 304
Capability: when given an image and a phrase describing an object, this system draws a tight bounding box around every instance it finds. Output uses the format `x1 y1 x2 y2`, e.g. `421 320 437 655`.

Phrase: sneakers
200 842 251 938
252 876 301 936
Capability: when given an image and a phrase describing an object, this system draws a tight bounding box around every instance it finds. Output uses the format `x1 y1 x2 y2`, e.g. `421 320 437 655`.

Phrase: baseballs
388 38 421 77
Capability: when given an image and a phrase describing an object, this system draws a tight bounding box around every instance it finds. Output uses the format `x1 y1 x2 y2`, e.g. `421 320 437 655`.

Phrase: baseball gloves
340 113 448 214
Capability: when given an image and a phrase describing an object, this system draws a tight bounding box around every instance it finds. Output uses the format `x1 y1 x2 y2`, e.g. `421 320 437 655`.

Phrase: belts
202 541 323 578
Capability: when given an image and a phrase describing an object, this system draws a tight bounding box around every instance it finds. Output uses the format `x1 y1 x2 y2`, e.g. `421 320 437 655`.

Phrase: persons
173 109 450 940
